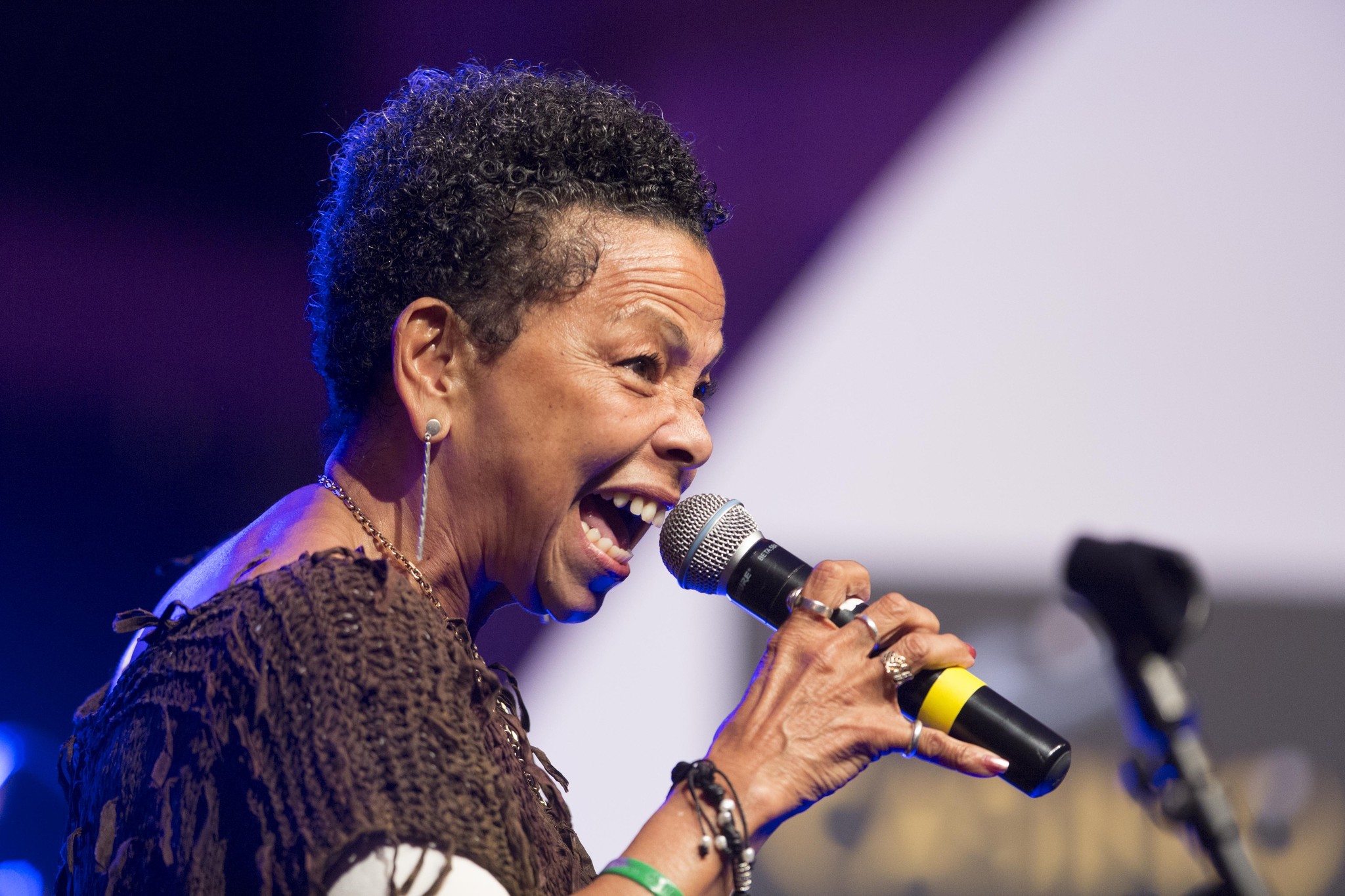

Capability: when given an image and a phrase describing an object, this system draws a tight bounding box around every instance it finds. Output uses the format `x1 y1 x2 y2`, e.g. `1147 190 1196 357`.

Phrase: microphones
659 493 1073 797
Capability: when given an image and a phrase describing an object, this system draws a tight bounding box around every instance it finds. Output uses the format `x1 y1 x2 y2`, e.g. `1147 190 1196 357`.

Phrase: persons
55 58 1010 896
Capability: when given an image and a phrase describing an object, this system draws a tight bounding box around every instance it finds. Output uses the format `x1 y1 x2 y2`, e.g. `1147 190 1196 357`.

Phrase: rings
792 594 833 619
902 718 923 760
855 613 880 646
880 651 914 690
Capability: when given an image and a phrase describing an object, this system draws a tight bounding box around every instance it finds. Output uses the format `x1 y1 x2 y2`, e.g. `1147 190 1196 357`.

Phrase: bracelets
663 760 756 896
600 858 684 896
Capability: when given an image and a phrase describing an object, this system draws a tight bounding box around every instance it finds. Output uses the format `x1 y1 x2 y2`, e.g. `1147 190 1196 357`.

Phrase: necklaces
317 474 548 808
